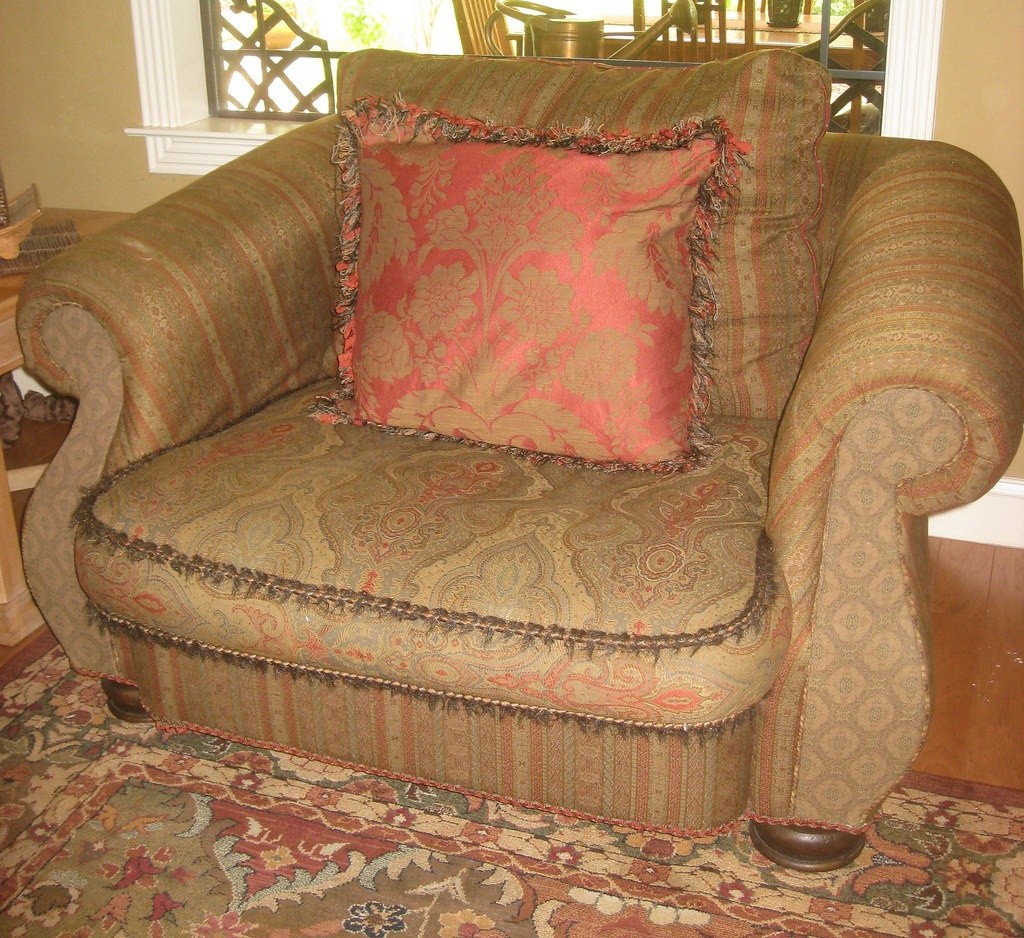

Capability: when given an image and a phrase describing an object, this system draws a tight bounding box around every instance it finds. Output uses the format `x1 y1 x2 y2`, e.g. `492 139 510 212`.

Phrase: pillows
333 95 750 469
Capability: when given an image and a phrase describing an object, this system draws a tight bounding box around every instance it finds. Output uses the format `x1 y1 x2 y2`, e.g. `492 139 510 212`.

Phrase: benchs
503 13 883 136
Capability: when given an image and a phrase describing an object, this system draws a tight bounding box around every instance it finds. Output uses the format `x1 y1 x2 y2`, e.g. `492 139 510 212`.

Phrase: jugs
483 0 698 60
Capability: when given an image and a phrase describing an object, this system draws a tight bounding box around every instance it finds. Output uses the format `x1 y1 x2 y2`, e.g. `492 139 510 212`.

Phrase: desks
0 208 140 616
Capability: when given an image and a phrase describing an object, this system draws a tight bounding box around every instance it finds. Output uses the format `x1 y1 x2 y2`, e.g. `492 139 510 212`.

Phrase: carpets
1 617 1024 938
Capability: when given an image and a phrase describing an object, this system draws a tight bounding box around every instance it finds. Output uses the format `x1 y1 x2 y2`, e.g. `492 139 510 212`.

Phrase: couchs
17 45 1023 869
